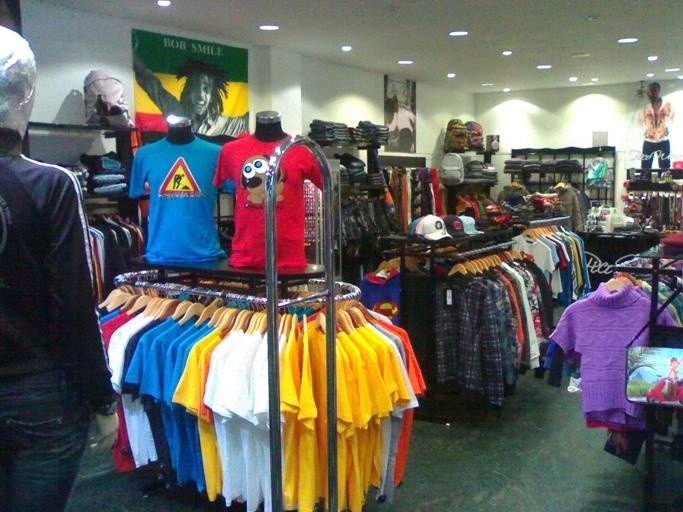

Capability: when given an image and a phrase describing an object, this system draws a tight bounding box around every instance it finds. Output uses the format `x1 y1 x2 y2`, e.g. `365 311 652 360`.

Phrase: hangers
371 223 570 280
92 271 375 337
599 250 672 300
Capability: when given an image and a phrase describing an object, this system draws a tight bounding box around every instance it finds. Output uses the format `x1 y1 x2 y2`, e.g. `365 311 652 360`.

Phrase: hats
405 213 485 243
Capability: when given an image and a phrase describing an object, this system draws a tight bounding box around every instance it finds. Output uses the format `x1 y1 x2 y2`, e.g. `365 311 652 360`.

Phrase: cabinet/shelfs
23 122 150 271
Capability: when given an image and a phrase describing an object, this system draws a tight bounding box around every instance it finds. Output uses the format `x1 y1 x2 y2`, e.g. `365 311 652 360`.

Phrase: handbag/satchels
624 345 683 410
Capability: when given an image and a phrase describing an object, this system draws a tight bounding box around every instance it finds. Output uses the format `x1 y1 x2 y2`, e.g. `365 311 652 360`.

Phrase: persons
640 81 672 177
211 107 327 271
132 47 250 138
125 114 228 261
1 23 123 512
383 93 417 152
665 356 683 400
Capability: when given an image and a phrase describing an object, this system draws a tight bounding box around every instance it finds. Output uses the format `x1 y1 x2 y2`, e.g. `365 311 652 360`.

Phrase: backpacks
463 120 484 152
438 152 464 187
80 67 135 130
443 118 468 154
